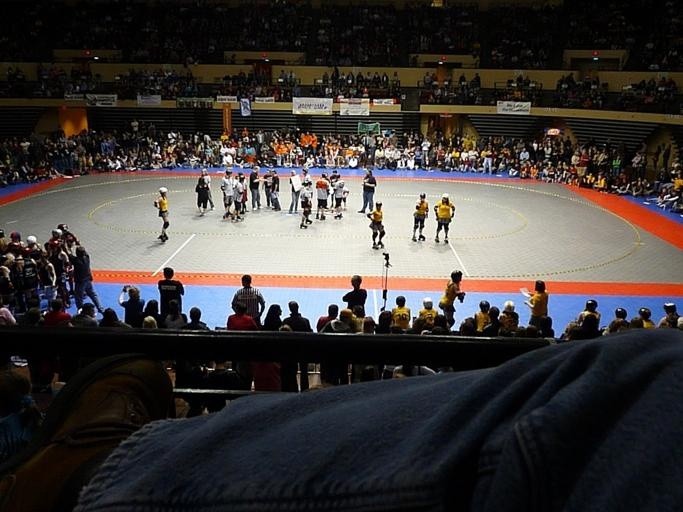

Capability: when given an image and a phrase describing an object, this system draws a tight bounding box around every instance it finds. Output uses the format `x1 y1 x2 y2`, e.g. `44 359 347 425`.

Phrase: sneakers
0 353 175 511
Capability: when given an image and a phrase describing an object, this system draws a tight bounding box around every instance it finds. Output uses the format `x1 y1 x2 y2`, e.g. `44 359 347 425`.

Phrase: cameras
458 295 464 303
124 285 131 290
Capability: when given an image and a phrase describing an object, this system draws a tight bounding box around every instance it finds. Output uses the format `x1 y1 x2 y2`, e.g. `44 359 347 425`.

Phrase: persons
1 370 44 462
1 119 683 213
365 201 386 247
153 186 171 243
300 178 314 229
221 169 244 221
433 192 456 242
1 221 556 420
559 299 683 339
412 192 428 242
2 0 682 114
1 326 680 512
